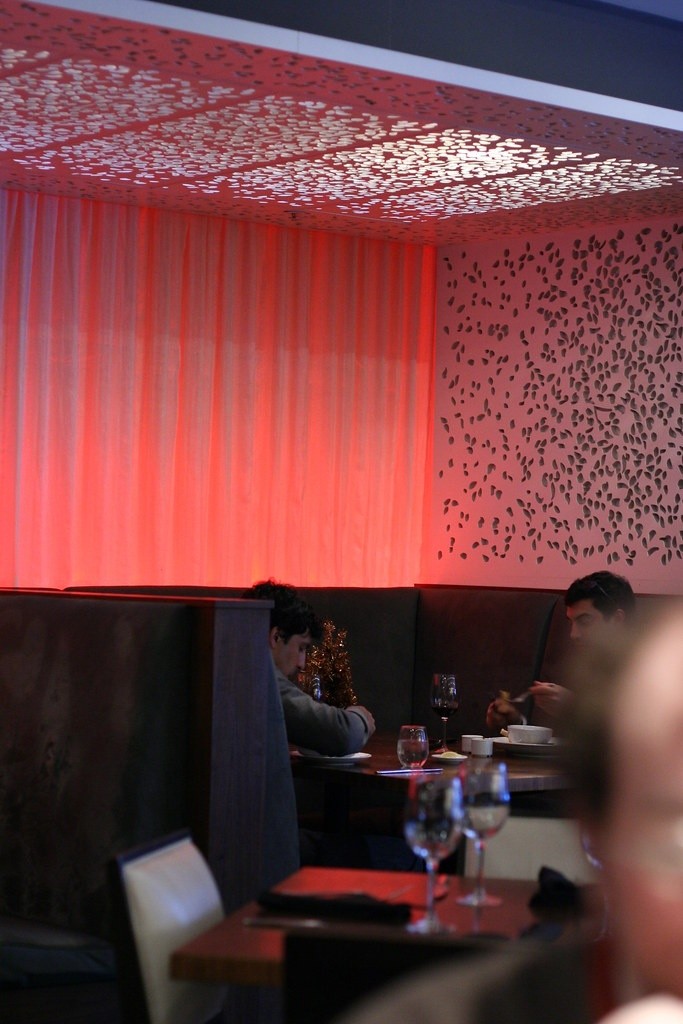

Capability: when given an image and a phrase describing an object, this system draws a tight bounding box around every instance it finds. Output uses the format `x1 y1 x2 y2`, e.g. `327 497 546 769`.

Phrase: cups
293 668 321 701
396 725 428 767
462 735 482 752
471 737 492 757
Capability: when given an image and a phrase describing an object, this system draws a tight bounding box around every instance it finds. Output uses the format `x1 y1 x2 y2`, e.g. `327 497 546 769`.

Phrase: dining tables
287 728 605 867
167 863 609 1024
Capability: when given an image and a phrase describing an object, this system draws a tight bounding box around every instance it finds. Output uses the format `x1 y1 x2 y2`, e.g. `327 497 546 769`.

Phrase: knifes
376 768 442 774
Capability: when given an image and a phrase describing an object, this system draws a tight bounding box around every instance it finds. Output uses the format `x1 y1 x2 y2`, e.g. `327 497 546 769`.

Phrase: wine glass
453 762 511 906
431 674 459 756
406 774 462 934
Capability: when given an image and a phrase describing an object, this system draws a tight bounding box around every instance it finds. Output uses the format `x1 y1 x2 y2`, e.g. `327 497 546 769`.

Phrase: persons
238 575 376 756
484 569 637 739
333 596 683 1023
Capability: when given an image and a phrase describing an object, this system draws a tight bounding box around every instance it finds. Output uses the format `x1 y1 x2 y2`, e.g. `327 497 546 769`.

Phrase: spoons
504 684 554 703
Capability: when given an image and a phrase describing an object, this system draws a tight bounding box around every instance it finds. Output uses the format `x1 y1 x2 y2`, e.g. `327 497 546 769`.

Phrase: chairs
284 921 515 1022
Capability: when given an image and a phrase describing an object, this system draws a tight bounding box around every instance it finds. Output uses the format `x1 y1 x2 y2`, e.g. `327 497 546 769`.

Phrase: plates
290 750 372 766
491 737 561 757
432 754 467 764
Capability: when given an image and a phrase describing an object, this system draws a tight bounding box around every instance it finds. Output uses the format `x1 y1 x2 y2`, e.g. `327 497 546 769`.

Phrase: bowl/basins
508 725 553 743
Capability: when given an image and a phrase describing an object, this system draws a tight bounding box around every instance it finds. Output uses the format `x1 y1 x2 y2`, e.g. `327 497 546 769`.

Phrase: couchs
0 582 300 993
258 581 683 750
